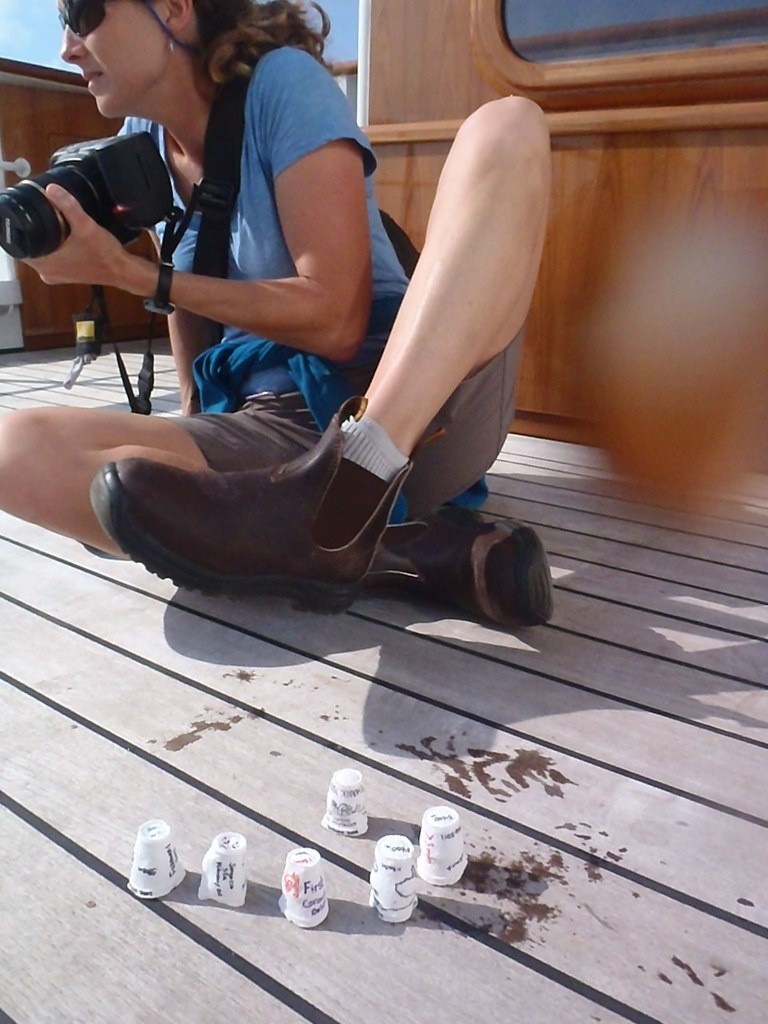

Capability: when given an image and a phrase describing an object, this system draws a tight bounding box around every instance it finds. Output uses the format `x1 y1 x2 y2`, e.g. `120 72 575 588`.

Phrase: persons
1 0 555 628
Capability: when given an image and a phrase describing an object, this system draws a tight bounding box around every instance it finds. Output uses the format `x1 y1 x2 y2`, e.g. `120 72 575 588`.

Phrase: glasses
58 0 109 37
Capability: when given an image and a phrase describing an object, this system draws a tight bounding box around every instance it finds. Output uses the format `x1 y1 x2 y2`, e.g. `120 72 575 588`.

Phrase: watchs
141 261 175 315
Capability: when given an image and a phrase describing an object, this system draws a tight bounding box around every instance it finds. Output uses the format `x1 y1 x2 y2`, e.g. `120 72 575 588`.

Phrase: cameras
0 132 173 260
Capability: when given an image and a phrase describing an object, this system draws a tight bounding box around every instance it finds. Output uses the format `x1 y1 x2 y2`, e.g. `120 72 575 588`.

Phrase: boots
91 397 412 617
364 504 552 629
